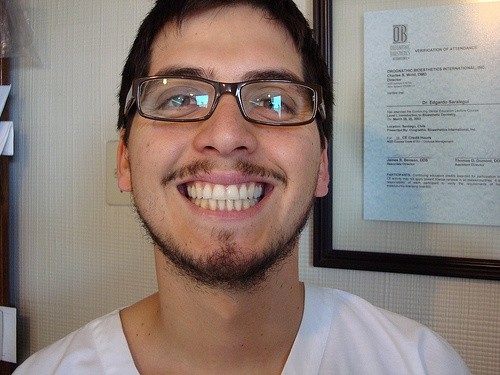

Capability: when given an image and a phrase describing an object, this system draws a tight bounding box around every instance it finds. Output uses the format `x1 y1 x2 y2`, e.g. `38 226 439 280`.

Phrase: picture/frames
313 2 500 282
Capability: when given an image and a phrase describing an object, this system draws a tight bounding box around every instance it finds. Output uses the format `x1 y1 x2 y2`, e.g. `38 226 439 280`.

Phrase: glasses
123 75 327 126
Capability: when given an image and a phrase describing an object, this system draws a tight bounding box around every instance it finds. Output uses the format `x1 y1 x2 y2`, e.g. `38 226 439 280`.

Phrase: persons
11 0 473 375
